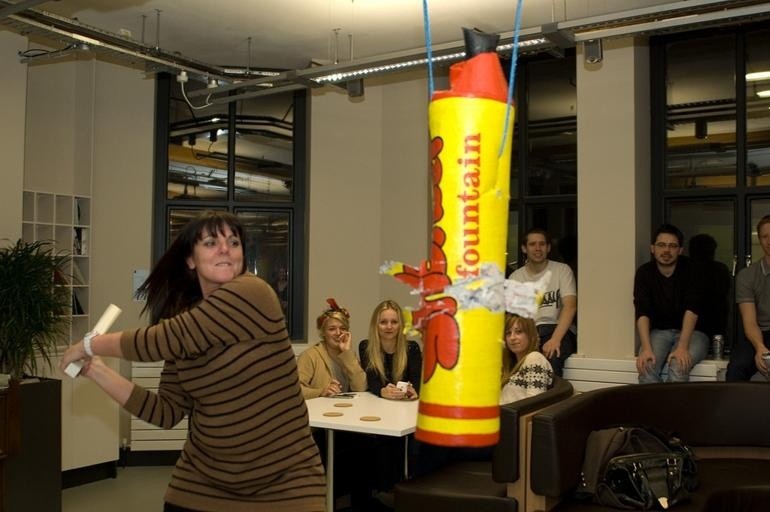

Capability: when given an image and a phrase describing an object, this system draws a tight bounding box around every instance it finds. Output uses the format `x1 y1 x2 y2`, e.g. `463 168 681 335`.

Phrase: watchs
82 331 98 357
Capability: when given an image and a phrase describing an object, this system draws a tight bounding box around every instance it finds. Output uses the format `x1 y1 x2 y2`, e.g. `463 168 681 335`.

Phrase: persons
506 229 577 378
61 211 327 512
688 233 729 347
358 300 422 490
296 299 372 512
499 315 554 405
727 215 770 382
634 225 710 383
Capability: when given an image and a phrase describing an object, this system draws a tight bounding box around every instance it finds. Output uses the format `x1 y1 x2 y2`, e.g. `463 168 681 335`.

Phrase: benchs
394 374 573 512
530 382 770 512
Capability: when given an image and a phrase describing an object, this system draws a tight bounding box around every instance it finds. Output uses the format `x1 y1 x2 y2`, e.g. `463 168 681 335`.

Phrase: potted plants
0 236 86 390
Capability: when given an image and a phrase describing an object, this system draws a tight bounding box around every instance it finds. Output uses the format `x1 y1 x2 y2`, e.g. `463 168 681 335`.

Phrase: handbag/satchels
576 427 699 511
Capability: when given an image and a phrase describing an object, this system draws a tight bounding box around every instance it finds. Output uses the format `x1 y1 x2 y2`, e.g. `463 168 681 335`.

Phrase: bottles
712 334 724 361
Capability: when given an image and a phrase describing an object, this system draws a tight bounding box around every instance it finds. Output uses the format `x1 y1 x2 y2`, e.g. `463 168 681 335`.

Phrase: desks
306 388 419 512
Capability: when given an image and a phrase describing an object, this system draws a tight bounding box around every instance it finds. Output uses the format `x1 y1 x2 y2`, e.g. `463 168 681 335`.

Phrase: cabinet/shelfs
21 189 92 359
